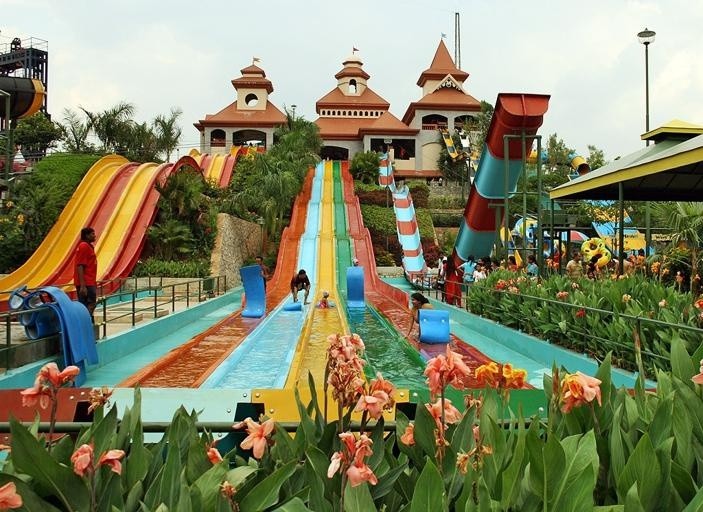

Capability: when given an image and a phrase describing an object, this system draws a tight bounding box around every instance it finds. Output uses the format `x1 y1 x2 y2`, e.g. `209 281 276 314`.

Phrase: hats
323 291 328 296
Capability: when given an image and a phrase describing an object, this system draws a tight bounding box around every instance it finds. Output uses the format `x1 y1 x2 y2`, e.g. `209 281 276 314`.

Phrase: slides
111 165 316 389
0 144 266 322
439 128 655 269
340 160 538 391
379 157 437 290
283 161 369 390
198 160 326 389
377 93 551 306
331 160 457 391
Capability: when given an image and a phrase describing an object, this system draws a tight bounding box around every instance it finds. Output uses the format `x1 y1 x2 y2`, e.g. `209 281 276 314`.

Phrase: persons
318 291 328 308
399 146 408 159
253 255 270 297
290 269 309 306
403 292 432 340
73 227 96 321
434 247 645 308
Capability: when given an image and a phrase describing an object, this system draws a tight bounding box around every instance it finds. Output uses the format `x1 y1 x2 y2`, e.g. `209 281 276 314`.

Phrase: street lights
176 148 180 160
635 26 658 261
607 231 616 259
291 104 297 122
382 136 393 209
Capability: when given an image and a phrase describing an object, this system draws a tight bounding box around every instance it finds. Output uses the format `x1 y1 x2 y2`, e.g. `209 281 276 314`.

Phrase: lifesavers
580 237 611 269
